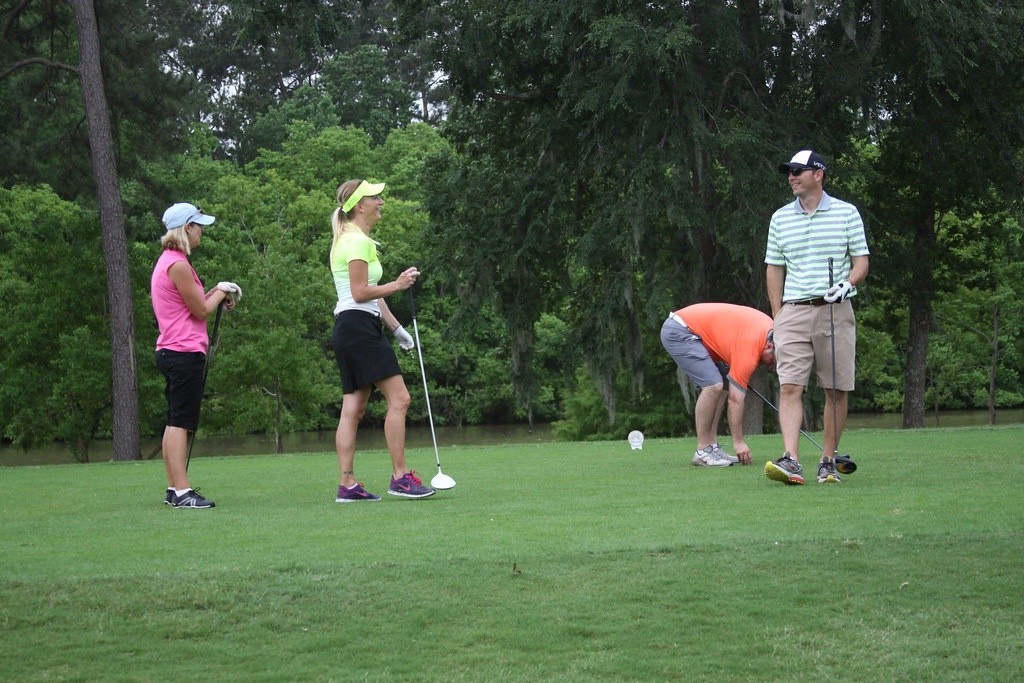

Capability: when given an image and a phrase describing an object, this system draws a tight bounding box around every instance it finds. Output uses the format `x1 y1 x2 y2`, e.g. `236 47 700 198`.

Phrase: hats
778 149 827 180
341 179 386 214
161 202 216 230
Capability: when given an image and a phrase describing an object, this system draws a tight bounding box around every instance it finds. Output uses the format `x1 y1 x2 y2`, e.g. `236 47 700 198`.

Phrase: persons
660 303 777 468
150 203 242 508
764 150 870 485
330 180 437 502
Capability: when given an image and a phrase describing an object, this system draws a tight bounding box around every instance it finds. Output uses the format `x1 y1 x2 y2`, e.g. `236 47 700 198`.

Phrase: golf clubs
716 359 851 465
185 298 227 476
827 256 858 475
405 283 457 490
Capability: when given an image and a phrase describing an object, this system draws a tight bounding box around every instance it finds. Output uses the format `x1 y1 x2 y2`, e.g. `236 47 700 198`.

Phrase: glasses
786 167 817 179
186 204 206 224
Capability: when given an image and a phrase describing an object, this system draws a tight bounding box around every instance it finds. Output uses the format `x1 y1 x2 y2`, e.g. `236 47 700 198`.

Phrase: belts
668 312 694 334
789 295 842 306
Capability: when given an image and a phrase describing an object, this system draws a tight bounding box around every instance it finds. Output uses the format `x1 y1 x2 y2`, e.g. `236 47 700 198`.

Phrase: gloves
217 281 243 301
824 280 854 303
393 324 415 351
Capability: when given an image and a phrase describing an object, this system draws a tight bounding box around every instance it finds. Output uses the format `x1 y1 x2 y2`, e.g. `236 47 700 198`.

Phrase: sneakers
692 445 735 468
713 442 739 462
336 481 382 503
816 454 841 484
170 487 216 509
765 449 805 485
386 468 436 498
165 487 173 506
